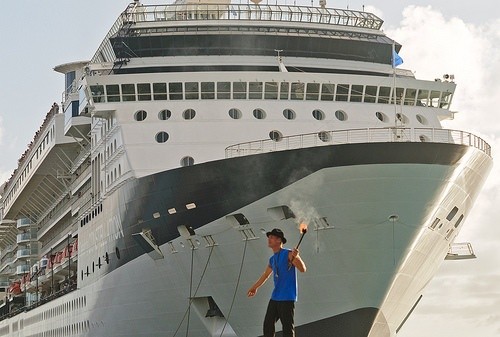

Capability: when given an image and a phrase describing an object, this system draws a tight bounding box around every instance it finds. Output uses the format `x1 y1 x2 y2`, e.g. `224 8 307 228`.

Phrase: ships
0 0 494 336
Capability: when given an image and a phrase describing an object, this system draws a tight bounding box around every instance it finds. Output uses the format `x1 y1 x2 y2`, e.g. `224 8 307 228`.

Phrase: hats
266 228 287 244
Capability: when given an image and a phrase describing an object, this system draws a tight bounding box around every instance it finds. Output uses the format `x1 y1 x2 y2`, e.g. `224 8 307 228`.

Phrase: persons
247 228 307 337
